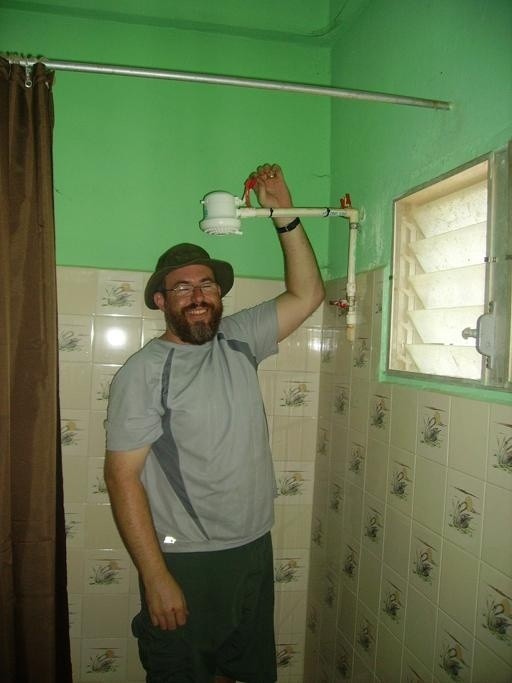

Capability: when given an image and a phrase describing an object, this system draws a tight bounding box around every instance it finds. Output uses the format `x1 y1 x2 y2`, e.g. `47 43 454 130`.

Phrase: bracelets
276 216 301 235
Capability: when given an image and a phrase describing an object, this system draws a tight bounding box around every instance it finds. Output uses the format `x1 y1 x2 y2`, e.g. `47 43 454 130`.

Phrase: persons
101 163 328 683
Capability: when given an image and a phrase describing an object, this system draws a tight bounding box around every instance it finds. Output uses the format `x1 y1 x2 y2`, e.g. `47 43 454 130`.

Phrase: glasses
161 281 217 298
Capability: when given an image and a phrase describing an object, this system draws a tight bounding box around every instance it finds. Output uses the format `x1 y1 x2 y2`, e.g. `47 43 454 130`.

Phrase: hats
144 243 235 310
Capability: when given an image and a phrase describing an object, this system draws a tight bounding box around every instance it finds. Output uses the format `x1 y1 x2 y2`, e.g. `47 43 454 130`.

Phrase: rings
269 174 277 181
